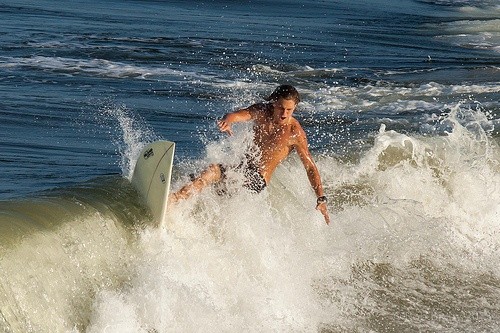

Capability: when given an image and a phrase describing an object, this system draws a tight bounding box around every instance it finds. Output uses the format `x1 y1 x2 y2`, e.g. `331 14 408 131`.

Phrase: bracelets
316 196 327 210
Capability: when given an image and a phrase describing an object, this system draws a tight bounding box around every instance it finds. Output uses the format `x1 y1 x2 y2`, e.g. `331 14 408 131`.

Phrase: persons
166 84 329 225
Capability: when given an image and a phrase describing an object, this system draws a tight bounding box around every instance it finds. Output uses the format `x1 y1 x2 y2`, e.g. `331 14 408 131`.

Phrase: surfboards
132 141 175 228
